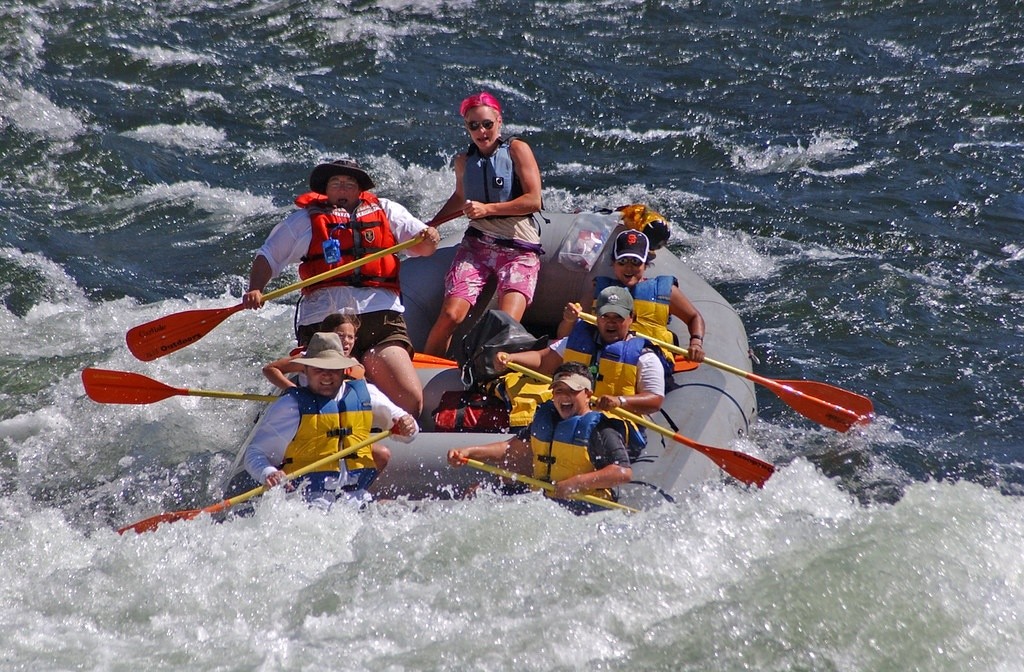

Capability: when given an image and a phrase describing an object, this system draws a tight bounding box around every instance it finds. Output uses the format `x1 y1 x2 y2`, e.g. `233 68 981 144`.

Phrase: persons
423 92 541 358
243 313 418 509
557 229 705 378
243 159 440 419
447 361 633 517
493 286 664 463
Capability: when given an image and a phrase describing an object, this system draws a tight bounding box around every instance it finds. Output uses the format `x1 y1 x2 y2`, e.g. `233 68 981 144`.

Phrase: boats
217 215 756 508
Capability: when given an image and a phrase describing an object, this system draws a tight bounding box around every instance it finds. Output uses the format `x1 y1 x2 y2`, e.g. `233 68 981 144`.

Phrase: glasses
464 117 496 130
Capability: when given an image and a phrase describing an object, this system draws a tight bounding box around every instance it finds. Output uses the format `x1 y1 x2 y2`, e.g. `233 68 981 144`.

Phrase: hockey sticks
445 446 645 516
498 352 777 490
117 422 412 542
568 302 880 442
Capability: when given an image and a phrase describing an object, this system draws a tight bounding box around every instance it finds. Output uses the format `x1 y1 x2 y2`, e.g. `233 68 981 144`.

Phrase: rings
410 425 412 428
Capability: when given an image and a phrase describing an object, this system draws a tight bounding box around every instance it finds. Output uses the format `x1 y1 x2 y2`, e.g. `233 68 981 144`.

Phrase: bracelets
689 335 703 341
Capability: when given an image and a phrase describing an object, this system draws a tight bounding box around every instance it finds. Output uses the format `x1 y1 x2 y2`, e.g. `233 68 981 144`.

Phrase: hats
612 229 650 263
310 156 376 192
595 286 635 320
547 372 591 391
288 332 358 370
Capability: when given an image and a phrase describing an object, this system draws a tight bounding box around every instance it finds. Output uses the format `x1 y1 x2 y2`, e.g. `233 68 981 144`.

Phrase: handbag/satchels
432 387 510 434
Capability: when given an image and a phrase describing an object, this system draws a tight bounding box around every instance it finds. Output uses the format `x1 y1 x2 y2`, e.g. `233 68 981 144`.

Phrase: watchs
618 396 626 408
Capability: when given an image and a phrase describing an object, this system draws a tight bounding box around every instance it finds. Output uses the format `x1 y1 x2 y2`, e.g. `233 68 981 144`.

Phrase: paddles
124 231 432 361
79 368 284 406
